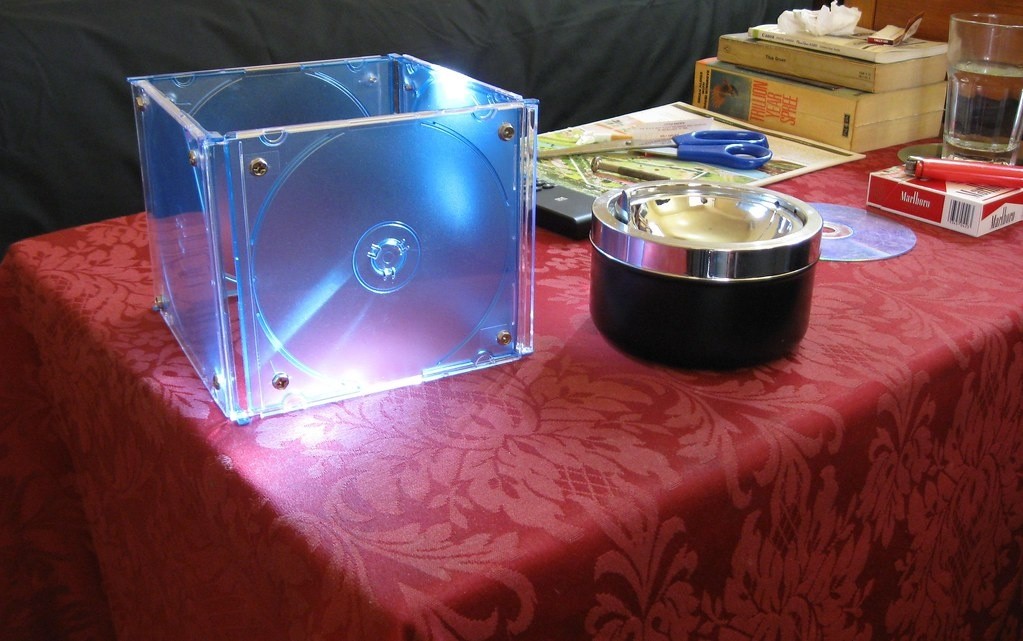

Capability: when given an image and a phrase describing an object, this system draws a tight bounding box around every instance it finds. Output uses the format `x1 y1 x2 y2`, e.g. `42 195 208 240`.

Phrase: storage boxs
127 53 538 423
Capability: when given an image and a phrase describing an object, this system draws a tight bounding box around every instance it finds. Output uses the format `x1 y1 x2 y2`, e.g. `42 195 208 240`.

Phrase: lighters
905 155 1023 189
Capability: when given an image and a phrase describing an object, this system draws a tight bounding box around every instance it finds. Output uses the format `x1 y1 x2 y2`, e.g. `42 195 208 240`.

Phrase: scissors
519 129 773 171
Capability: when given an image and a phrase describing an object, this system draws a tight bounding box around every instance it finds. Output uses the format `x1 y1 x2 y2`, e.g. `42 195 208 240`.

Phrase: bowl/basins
589 179 822 370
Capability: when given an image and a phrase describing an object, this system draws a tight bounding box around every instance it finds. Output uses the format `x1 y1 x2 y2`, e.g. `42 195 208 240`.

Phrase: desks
0 98 1023 640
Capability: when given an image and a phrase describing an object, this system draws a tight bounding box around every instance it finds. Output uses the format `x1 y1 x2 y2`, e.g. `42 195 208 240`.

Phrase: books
692 56 949 153
749 23 949 63
717 33 947 93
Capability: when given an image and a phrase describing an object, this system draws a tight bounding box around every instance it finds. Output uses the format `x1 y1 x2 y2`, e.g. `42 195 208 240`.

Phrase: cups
941 13 1023 166
